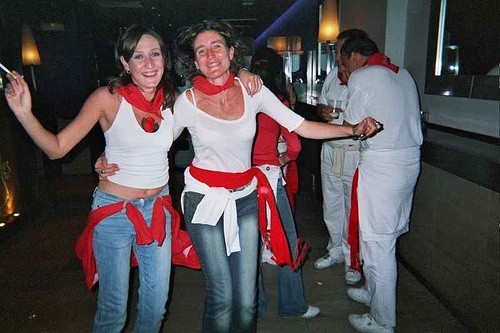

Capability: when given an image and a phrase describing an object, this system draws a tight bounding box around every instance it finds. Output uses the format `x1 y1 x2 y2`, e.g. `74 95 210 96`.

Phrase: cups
327 99 342 119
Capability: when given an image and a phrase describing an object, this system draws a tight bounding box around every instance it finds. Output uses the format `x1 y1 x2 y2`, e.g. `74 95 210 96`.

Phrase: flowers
142 116 159 133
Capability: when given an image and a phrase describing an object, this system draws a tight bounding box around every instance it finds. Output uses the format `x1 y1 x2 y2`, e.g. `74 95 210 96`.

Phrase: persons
315 29 368 284
95 20 382 333
5 25 263 333
250 47 320 317
341 35 424 333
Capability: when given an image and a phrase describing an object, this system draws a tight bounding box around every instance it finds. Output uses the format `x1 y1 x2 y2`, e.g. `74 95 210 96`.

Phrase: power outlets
420 112 430 122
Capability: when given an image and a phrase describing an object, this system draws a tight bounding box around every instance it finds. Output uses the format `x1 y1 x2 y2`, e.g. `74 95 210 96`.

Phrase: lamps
20 21 41 93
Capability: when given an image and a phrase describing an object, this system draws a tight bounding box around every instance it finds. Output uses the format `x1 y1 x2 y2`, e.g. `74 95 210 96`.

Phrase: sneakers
284 305 320 319
347 286 372 307
314 253 345 269
345 266 361 284
349 312 394 333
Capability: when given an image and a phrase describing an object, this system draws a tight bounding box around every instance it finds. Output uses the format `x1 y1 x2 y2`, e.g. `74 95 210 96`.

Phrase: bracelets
333 109 335 112
352 125 356 136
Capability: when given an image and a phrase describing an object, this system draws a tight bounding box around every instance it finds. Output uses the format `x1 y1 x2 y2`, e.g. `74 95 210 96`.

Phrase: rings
101 169 104 173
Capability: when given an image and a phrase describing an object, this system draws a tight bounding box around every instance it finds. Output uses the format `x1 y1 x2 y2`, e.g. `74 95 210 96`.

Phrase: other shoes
261 247 277 266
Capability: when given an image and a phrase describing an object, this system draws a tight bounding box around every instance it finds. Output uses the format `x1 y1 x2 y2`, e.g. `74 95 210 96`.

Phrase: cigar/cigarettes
0 63 16 80
